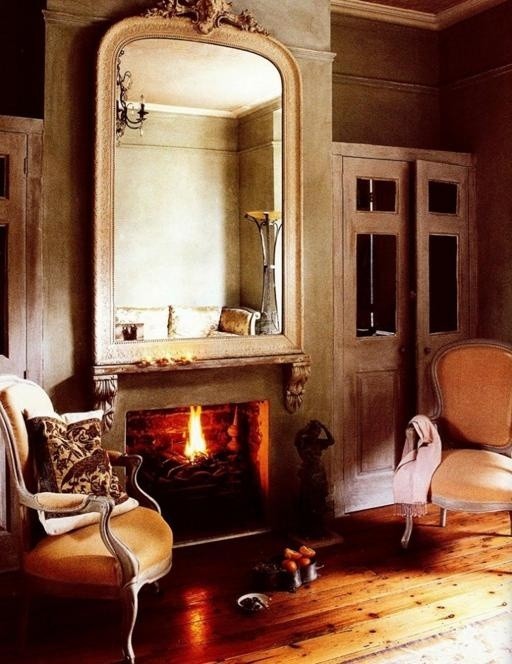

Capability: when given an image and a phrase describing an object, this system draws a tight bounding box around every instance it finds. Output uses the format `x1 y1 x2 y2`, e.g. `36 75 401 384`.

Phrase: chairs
0 374 177 663
399 336 512 550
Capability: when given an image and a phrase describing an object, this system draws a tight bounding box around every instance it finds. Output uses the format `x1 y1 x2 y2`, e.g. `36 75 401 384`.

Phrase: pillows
16 405 140 540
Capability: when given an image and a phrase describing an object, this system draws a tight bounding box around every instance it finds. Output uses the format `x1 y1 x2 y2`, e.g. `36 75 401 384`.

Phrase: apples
281 546 317 572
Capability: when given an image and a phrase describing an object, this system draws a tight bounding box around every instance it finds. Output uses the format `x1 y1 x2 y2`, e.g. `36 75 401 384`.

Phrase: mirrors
90 0 306 365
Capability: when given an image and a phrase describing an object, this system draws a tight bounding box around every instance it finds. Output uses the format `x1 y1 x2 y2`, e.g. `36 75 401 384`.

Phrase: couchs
113 303 261 341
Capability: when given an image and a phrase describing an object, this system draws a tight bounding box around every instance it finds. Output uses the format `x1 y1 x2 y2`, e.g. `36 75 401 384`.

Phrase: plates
236 592 272 612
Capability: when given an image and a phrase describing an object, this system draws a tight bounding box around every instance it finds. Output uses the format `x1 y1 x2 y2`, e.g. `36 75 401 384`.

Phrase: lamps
242 210 282 335
116 48 149 147
